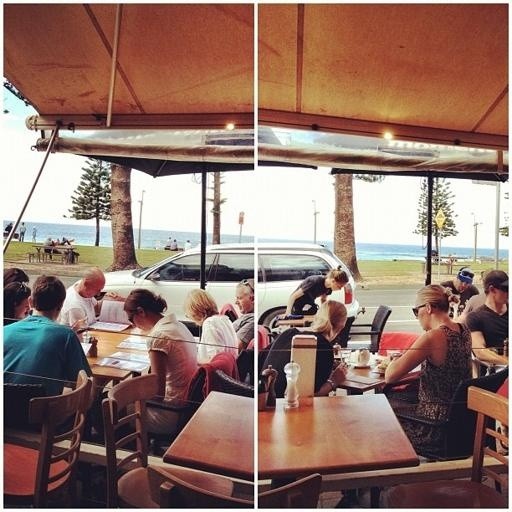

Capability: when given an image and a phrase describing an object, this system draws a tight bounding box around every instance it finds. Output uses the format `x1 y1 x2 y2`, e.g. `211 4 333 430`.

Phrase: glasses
412 304 437 316
127 311 142 325
242 278 254 290
13 281 28 307
458 270 474 279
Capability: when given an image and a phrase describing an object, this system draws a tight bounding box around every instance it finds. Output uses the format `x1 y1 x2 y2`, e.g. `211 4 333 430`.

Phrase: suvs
82 240 366 348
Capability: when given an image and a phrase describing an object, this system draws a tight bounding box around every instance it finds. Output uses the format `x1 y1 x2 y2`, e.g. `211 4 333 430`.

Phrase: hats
456 266 474 284
483 270 508 290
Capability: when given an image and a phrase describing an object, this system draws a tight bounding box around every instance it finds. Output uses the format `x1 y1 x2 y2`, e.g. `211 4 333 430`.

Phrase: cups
386 348 400 362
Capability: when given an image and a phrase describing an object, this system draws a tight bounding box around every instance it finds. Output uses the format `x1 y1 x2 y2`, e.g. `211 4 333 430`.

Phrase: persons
285 265 350 316
4 267 93 432
186 289 237 362
258 300 349 398
232 279 254 354
384 285 473 442
57 266 120 331
123 290 198 436
440 268 508 380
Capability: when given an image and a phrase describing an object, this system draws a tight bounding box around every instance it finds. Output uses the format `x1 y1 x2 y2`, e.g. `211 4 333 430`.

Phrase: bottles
80 328 97 358
258 364 278 408
350 350 357 363
283 361 301 409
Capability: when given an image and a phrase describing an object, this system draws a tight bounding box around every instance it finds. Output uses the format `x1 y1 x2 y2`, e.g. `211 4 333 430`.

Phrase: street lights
309 198 321 242
472 211 479 262
136 188 146 249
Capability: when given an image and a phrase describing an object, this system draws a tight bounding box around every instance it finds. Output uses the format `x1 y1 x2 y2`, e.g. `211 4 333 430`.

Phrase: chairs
4 304 254 509
258 304 509 508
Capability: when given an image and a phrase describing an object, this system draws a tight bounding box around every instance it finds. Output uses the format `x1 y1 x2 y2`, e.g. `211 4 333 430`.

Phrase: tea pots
354 347 370 365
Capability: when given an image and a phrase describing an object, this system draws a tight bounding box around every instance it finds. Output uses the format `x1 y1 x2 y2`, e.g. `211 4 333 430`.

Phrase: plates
352 364 370 368
371 364 386 374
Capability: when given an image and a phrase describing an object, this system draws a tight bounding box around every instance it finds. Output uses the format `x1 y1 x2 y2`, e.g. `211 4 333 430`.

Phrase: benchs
423 256 469 274
28 246 78 262
